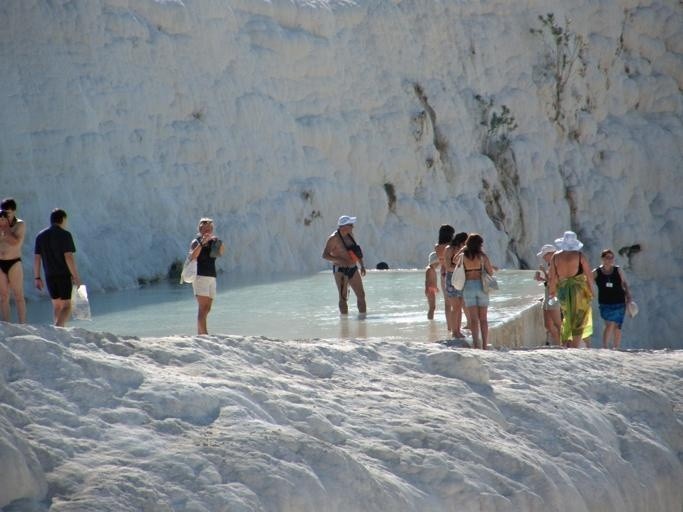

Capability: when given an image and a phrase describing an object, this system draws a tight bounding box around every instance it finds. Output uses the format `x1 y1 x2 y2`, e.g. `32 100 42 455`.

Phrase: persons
32 208 80 327
0 198 27 325
187 217 224 336
424 224 496 350
533 244 563 346
320 215 368 316
589 248 634 349
548 230 595 348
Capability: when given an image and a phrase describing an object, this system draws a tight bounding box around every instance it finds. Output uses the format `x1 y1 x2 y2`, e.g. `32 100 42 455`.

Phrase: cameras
349 243 362 259
0 210 8 217
208 238 222 249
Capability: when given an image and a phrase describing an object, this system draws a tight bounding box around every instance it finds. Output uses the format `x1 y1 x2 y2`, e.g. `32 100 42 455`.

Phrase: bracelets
199 241 203 248
360 263 365 269
33 276 40 281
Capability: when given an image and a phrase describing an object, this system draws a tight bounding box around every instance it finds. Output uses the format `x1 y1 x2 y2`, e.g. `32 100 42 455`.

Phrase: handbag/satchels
481 270 498 292
181 238 199 283
452 253 466 291
349 245 362 261
72 283 92 322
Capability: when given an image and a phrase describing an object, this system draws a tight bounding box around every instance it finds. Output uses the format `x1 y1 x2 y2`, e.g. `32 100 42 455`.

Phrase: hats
628 301 639 318
209 239 225 259
555 231 583 252
537 244 555 257
428 251 439 265
337 216 356 226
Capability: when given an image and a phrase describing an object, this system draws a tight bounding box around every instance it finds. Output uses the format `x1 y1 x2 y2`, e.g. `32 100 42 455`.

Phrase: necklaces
602 265 612 273
